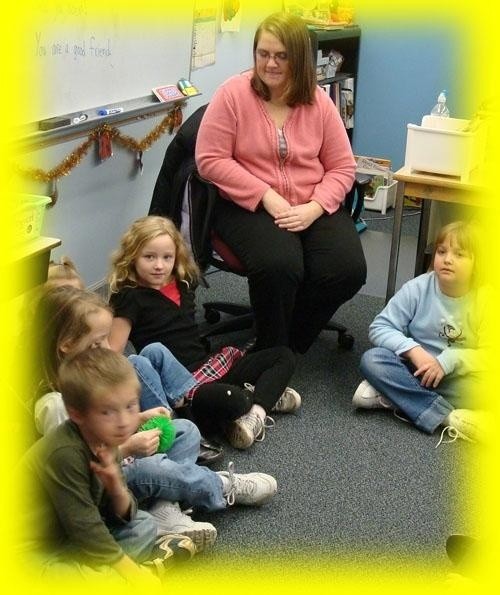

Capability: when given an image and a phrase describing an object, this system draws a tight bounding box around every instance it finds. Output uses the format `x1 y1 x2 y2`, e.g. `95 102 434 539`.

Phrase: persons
22 283 279 555
105 215 303 449
193 12 369 352
10 347 199 591
352 219 482 445
43 257 227 462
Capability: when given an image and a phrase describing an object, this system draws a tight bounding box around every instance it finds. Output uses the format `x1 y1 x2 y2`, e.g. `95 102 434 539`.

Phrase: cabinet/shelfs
309 25 362 148
9 237 64 308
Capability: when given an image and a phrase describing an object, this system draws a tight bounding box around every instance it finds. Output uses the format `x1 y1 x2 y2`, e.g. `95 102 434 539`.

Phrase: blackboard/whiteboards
11 2 198 147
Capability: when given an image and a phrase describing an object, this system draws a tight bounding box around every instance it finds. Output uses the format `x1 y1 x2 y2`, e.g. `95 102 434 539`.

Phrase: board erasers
41 117 70 130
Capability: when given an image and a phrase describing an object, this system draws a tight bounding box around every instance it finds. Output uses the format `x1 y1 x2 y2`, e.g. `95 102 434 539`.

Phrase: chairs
178 103 370 351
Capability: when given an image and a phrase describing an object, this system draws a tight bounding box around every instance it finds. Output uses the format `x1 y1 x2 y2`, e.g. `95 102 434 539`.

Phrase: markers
74 114 88 123
98 107 123 116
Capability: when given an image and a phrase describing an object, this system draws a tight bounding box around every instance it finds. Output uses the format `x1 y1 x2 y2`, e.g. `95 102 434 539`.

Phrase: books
316 49 394 198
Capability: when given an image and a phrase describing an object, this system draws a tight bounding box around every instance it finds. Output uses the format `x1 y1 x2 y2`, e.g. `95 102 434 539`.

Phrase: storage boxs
21 194 52 250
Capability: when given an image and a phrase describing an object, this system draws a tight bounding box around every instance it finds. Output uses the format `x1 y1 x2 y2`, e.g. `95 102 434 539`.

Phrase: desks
383 167 482 312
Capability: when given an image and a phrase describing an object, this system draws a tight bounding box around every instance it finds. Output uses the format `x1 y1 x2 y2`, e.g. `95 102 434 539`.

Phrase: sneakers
150 506 217 553
216 471 277 506
143 533 196 577
450 408 480 441
351 379 399 410
244 385 301 412
224 414 264 449
446 533 475 564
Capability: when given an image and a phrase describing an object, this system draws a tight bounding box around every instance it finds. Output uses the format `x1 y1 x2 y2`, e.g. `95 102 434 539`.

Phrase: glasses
255 49 288 62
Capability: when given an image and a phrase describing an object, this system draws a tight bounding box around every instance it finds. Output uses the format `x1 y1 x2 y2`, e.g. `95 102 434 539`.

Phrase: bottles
430 91 449 129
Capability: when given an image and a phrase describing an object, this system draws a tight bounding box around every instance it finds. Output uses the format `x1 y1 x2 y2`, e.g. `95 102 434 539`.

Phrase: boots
173 404 223 466
